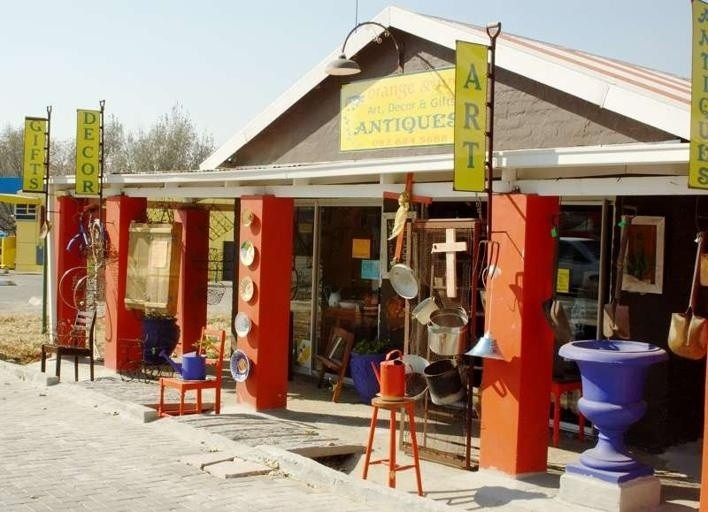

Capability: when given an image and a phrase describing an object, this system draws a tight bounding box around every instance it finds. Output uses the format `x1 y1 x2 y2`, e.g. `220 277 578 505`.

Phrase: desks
322 304 378 349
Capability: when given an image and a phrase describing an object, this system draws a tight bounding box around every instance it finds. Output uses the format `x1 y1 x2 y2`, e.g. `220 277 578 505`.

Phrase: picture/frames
620 215 665 295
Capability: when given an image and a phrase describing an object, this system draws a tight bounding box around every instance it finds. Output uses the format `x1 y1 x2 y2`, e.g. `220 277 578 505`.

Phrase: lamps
324 21 404 78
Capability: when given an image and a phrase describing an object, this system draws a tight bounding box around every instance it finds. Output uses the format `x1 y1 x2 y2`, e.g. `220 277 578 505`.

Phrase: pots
409 296 441 326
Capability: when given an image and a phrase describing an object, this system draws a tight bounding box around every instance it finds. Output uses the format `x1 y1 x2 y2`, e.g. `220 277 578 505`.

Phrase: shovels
668 232 708 359
603 215 630 340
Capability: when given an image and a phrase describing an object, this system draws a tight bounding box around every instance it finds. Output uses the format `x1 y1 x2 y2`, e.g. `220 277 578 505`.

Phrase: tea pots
324 285 343 308
371 348 414 402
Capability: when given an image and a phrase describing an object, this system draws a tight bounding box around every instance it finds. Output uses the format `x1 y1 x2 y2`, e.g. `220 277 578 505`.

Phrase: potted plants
351 337 391 404
142 310 180 364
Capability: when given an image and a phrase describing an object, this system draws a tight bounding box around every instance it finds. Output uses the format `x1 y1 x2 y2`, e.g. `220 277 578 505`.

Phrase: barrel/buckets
426 308 469 357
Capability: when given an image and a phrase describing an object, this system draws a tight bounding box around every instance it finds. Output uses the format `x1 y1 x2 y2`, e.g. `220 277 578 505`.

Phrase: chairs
41 310 97 381
158 326 226 417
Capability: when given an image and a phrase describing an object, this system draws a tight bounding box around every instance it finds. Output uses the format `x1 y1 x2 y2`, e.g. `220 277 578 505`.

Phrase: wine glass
558 337 669 474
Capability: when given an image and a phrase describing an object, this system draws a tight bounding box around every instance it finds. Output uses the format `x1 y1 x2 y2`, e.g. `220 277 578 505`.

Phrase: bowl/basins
230 207 256 383
339 301 357 309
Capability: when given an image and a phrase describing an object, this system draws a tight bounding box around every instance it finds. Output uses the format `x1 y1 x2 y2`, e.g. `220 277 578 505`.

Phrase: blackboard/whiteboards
380 211 417 280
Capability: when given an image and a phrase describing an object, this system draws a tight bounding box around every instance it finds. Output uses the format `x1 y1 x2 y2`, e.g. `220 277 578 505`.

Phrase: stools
361 398 423 495
550 380 584 447
